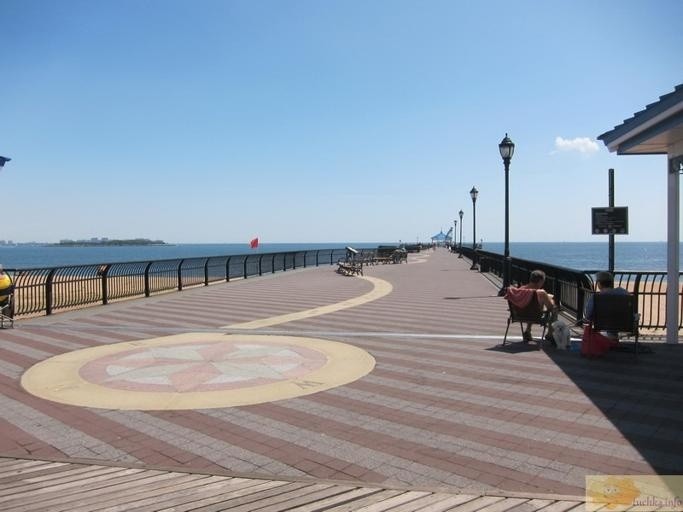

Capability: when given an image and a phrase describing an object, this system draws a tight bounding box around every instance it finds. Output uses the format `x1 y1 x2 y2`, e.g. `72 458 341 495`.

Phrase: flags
250 238 259 249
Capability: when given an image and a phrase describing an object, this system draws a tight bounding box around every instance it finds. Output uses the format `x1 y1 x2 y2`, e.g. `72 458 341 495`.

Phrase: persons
518 269 562 346
358 239 456 266
0 264 14 307
584 270 641 348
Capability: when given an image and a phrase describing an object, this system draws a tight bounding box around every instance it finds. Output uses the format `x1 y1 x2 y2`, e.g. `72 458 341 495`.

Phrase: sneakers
608 341 622 350
545 334 552 342
525 330 532 341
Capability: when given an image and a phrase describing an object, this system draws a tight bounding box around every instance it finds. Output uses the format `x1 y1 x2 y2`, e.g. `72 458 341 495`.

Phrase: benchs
336 247 408 278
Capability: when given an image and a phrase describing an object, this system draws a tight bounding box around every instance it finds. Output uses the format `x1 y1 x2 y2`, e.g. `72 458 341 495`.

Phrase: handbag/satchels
582 327 608 356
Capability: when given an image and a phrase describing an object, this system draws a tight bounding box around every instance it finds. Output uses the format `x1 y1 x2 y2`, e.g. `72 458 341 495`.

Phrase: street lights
447 208 464 259
468 184 480 271
498 133 514 297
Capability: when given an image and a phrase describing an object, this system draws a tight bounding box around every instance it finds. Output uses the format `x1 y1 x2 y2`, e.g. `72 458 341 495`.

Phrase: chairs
588 288 641 352
501 285 559 353
